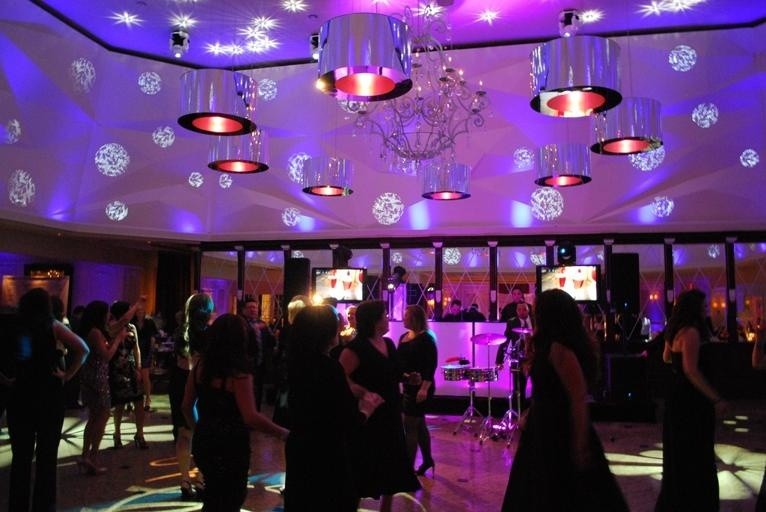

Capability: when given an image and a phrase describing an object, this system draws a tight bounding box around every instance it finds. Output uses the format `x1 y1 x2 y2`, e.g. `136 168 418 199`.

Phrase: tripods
475 383 504 445
453 382 485 436
489 374 523 447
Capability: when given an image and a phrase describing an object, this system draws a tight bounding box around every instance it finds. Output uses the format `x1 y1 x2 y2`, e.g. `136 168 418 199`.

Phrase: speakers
608 253 641 314
284 258 310 311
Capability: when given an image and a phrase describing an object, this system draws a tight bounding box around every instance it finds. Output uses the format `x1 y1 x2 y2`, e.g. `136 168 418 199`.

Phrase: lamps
176 69 258 136
348 4 491 168
302 157 354 196
168 25 191 59
589 97 664 156
207 129 270 174
533 143 592 186
314 13 412 102
421 162 472 201
528 11 623 118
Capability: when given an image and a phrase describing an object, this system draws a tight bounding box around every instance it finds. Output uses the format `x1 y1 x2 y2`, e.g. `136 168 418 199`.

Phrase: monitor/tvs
312 267 367 303
536 264 602 304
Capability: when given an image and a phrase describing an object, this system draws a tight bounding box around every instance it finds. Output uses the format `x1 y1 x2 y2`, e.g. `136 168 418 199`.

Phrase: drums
470 367 498 381
441 365 469 381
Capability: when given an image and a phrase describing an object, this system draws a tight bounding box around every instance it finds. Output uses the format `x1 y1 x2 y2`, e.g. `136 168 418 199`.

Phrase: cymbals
470 333 507 345
512 327 534 333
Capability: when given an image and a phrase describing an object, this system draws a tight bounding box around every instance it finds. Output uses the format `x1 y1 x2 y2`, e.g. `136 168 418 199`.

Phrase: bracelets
714 397 722 405
756 328 766 338
754 342 764 348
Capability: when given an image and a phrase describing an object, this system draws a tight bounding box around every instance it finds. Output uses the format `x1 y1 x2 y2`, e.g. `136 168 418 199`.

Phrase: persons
654 288 722 512
501 301 537 411
752 317 766 369
441 299 469 322
500 288 531 322
500 288 629 512
0 293 440 511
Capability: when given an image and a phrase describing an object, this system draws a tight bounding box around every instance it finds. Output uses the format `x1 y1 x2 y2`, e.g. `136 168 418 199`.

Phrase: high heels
78 458 108 475
134 435 148 449
415 459 434 476
181 480 204 499
113 433 122 449
